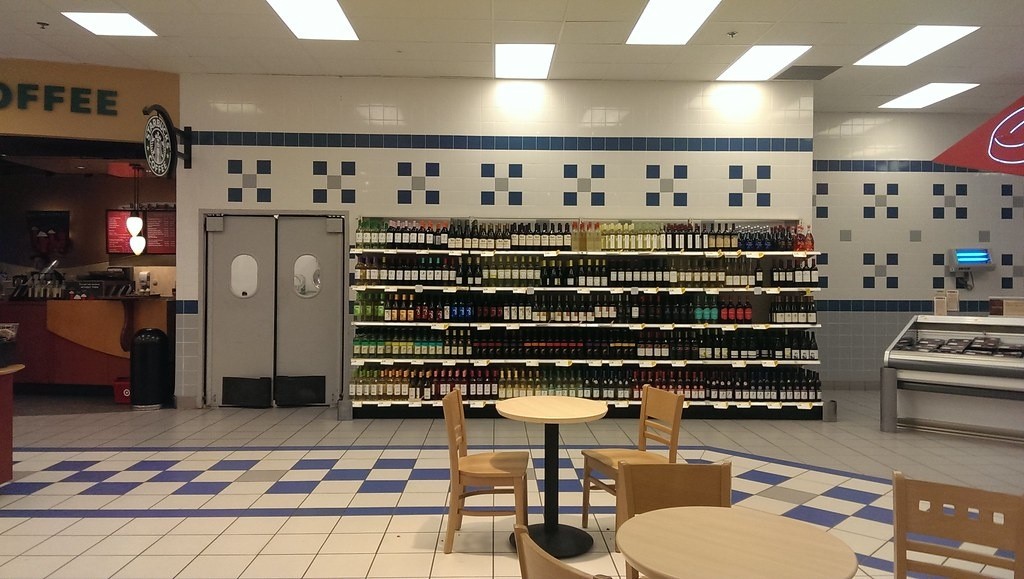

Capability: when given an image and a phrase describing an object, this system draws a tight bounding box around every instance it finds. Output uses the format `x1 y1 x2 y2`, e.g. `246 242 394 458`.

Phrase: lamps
126 163 144 238
130 167 147 256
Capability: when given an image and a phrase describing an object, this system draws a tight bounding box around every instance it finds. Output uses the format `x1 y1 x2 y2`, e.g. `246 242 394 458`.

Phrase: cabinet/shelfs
881 314 1024 444
348 247 826 406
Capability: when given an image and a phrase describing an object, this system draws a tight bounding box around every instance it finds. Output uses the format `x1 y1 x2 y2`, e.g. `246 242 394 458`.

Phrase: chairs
617 459 732 579
892 470 1024 579
513 524 613 579
580 383 685 552
442 388 529 554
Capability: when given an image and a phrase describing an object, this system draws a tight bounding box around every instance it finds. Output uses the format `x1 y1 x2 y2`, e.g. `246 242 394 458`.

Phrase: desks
615 505 859 579
494 396 608 558
0 363 26 487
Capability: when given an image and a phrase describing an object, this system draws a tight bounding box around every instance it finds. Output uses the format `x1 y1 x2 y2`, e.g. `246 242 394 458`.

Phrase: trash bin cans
131 328 169 410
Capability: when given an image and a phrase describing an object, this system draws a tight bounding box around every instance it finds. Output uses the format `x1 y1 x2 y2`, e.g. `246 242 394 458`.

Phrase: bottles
348 366 822 401
18 273 67 298
353 293 818 324
355 218 815 251
352 328 819 361
354 255 818 288
